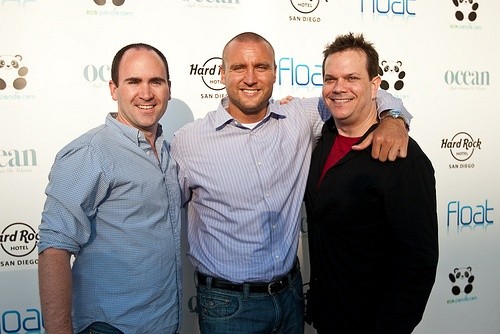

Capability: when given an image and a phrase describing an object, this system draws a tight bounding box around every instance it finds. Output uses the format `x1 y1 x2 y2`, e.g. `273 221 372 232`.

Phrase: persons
170 33 413 334
276 32 439 334
37 43 185 334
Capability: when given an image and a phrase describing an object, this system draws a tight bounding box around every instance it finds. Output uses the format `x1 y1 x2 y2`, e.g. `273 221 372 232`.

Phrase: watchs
381 108 410 132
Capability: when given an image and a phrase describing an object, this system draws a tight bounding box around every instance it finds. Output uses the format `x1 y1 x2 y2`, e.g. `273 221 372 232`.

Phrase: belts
196 256 301 296
